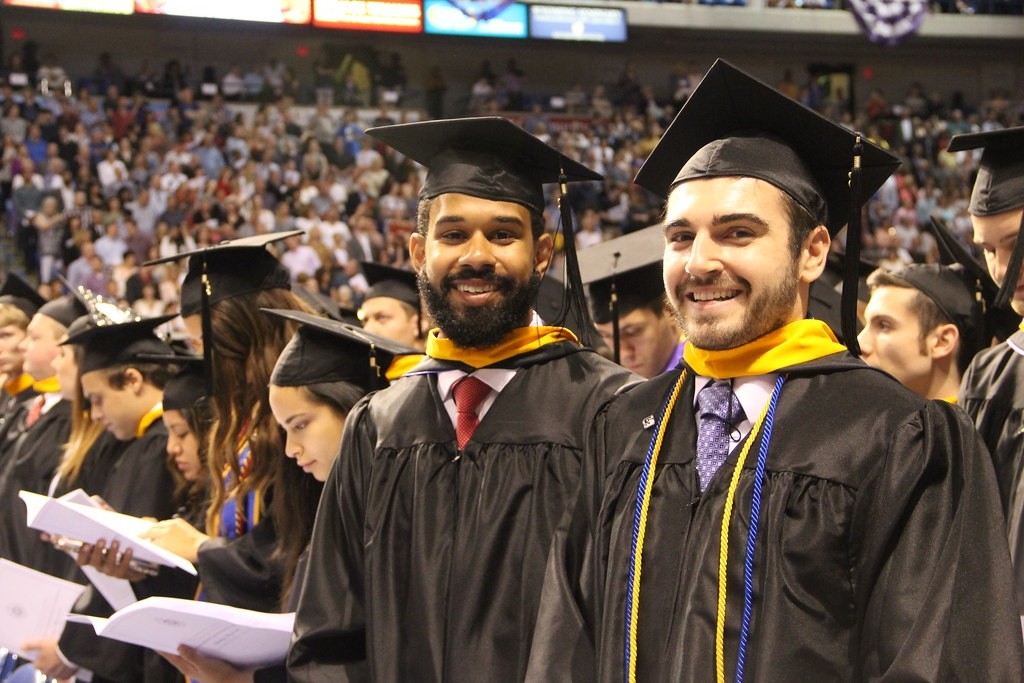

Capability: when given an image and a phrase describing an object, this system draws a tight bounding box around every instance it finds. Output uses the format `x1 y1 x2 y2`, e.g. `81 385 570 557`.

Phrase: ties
694 381 747 500
450 376 492 451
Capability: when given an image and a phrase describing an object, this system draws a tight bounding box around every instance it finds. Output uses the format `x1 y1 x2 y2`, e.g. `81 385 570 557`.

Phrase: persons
358 262 428 351
949 129 1023 619
287 117 648 683
0 230 326 682
855 215 997 403
572 225 684 378
524 57 1024 682
162 305 426 682
0 35 1022 322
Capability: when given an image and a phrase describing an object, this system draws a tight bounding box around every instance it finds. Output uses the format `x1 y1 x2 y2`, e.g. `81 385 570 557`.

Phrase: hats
0 58 1024 411
